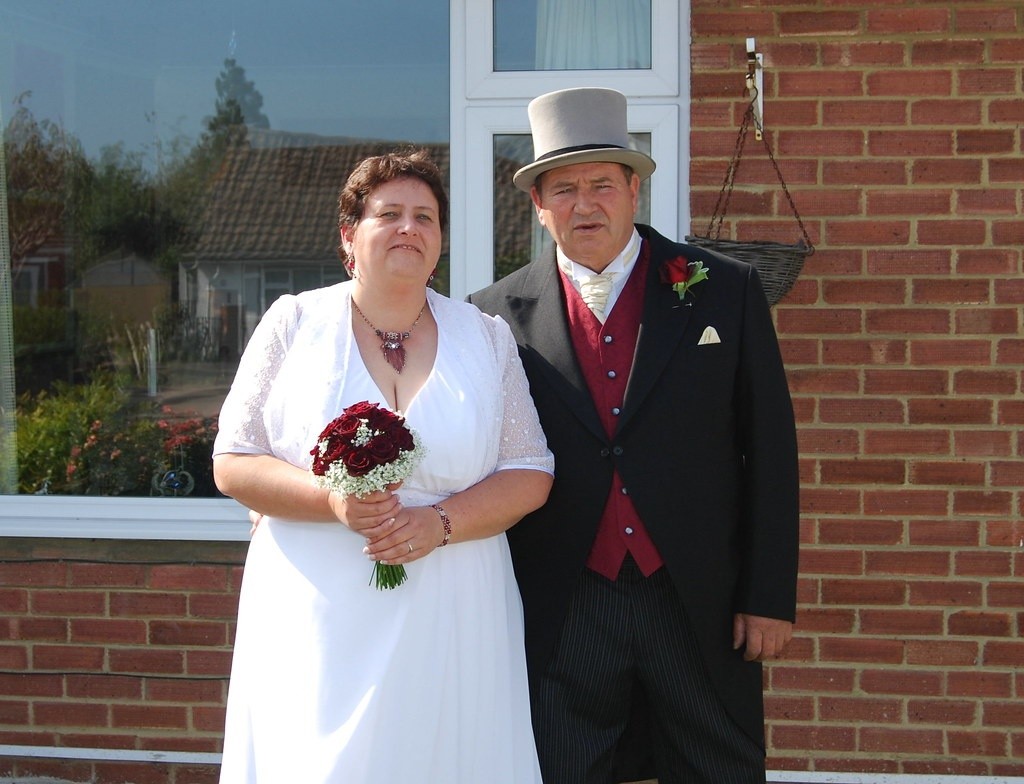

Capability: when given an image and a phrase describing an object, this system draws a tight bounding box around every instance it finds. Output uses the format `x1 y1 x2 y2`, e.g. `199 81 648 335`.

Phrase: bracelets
429 504 452 547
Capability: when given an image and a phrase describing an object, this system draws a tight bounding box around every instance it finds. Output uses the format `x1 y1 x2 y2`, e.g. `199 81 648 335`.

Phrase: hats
513 86 657 193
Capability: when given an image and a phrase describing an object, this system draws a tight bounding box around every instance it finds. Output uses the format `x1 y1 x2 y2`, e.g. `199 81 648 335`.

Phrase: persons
464 88 799 783
211 150 553 784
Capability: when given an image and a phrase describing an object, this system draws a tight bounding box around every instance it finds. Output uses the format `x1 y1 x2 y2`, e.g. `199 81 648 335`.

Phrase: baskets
684 233 816 307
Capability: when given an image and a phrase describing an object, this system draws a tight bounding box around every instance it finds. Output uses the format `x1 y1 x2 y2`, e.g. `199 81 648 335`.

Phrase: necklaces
350 294 427 374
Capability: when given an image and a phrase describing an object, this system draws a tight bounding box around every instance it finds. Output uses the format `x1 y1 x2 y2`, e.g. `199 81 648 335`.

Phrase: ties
577 275 611 327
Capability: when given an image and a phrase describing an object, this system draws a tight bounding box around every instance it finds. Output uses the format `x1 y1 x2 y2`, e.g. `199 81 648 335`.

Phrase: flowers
654 257 710 302
310 396 435 595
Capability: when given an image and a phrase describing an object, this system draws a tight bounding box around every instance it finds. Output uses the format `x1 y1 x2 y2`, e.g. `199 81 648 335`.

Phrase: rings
407 540 413 553
